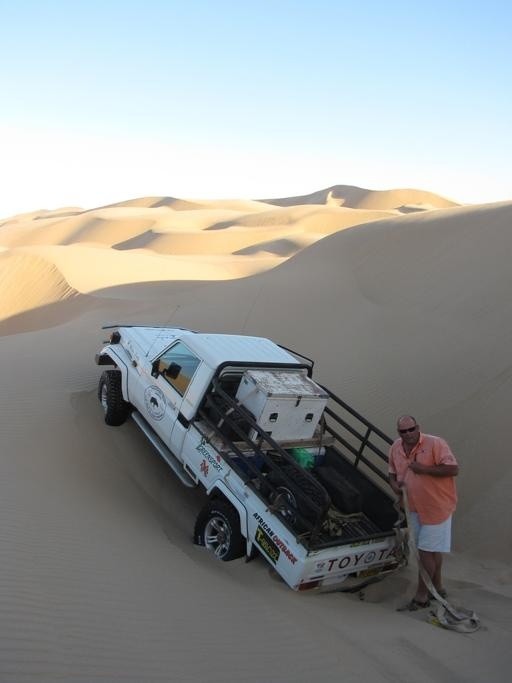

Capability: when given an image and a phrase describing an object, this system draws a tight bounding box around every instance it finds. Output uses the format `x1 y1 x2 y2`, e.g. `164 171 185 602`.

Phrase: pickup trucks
96 326 410 593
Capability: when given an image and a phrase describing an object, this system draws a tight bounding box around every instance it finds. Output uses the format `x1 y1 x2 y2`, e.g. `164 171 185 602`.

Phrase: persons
386 414 460 611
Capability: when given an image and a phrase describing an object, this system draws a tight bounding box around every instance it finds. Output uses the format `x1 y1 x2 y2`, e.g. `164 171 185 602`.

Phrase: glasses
399 425 416 434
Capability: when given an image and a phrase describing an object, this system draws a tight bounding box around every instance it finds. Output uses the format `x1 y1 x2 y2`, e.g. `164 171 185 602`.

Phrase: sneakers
427 589 448 601
396 598 430 613
